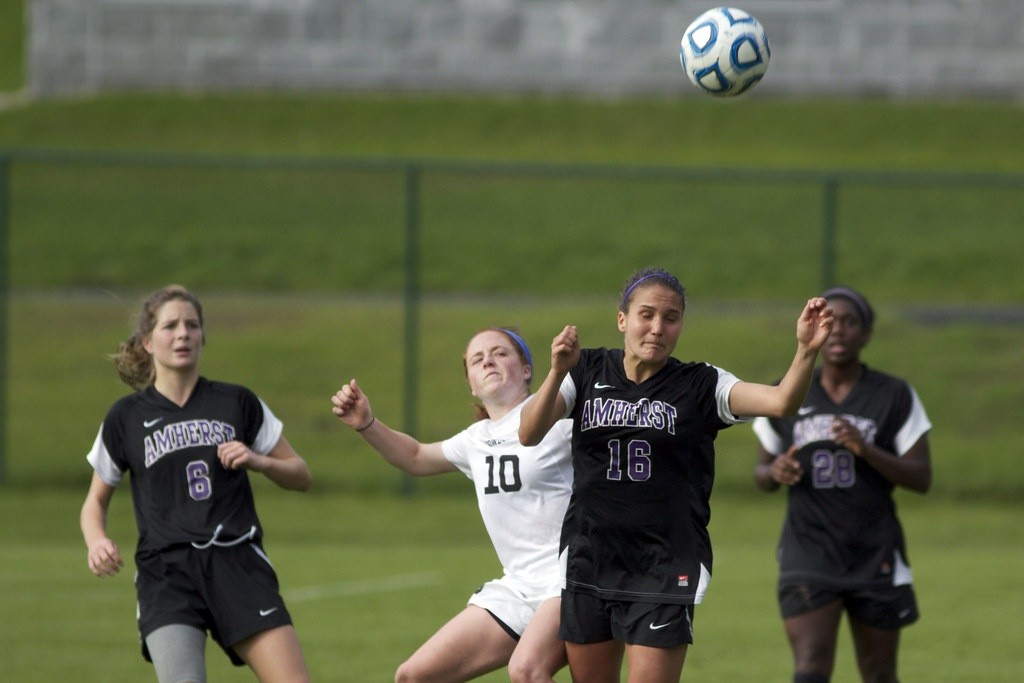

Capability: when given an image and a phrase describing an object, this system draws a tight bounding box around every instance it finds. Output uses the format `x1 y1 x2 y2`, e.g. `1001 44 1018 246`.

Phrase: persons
80 283 310 683
519 265 834 682
752 285 931 682
330 326 574 683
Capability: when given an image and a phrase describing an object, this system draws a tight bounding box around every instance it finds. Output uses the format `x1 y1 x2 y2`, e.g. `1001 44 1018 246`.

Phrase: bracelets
355 416 375 432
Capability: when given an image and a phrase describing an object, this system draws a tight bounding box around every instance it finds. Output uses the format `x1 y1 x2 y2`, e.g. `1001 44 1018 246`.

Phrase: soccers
679 7 771 98
